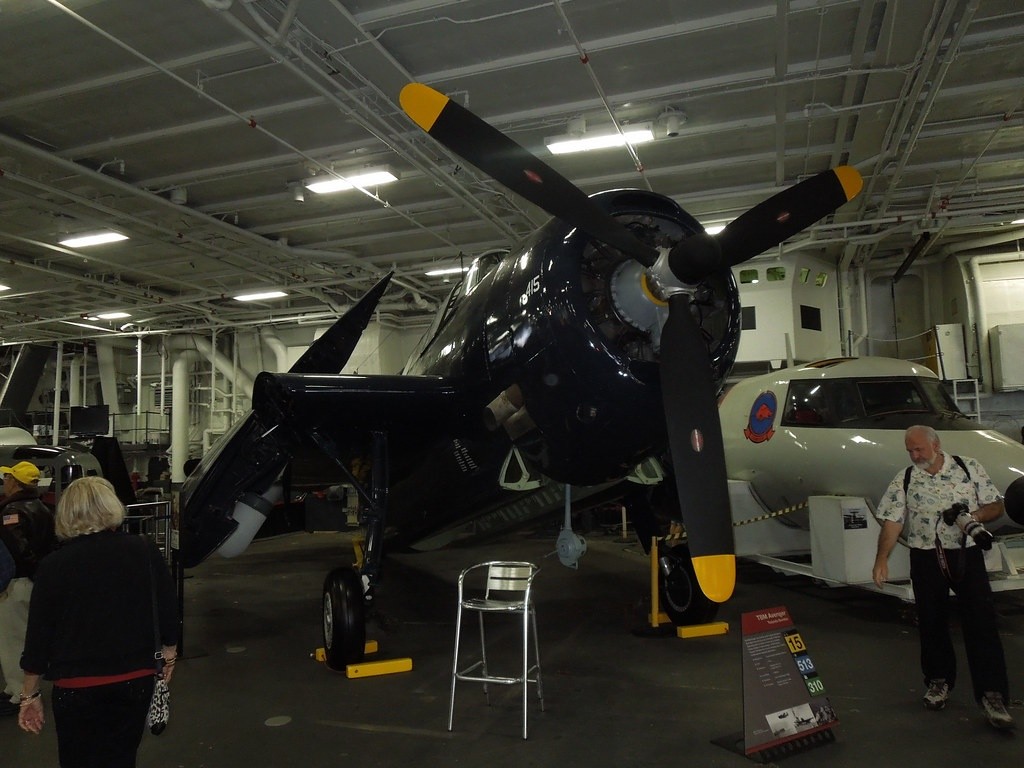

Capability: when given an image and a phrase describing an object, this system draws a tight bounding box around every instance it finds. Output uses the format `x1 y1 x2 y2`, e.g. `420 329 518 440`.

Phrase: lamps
543 120 654 155
301 163 400 195
56 228 130 248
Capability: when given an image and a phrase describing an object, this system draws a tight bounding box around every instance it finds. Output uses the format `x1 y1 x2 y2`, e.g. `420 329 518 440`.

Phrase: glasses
3 473 12 478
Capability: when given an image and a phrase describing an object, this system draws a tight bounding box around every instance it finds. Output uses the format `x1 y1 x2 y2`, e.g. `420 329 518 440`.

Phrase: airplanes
716 356 1024 538
179 82 863 674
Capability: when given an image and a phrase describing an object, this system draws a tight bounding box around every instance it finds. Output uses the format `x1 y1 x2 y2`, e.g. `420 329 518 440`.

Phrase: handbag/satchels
148 671 171 737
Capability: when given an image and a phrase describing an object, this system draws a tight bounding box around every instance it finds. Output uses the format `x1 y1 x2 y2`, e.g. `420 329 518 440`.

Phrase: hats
0 461 42 486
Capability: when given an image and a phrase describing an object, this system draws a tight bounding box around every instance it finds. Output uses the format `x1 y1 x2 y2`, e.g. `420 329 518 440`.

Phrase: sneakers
980 692 1016 730
923 678 954 710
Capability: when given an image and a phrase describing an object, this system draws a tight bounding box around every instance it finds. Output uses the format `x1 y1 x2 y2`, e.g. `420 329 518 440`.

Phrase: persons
0 462 182 768
871 423 1016 731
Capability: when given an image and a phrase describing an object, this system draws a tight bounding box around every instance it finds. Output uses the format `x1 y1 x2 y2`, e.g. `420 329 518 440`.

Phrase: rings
163 674 167 677
24 718 31 724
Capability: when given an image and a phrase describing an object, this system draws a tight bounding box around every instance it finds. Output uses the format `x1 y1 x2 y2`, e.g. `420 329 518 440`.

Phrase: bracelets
974 512 982 522
161 642 178 667
3 676 41 706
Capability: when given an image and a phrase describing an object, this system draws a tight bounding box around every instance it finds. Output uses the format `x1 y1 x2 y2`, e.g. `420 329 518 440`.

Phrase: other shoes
0 690 21 719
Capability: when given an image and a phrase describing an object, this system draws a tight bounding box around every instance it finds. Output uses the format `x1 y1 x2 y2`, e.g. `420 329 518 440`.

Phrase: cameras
943 502 994 553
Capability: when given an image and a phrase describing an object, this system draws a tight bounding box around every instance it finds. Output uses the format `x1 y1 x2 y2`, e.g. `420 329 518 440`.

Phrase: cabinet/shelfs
926 324 967 380
989 323 1024 392
953 379 980 424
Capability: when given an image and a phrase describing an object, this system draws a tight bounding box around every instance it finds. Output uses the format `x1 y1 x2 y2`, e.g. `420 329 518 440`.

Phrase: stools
448 561 545 739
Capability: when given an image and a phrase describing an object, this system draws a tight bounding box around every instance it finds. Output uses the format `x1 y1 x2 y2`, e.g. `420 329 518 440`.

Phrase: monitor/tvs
70 405 109 437
